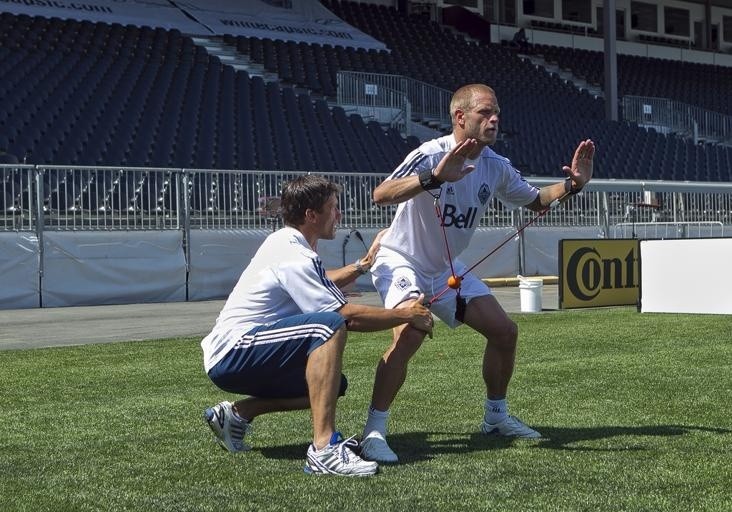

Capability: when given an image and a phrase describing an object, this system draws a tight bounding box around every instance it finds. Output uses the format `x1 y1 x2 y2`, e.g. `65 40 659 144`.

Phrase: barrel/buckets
519 279 543 312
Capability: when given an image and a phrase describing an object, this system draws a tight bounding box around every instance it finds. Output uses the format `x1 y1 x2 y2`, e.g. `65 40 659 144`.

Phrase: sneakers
359 425 399 463
482 415 542 438
303 433 379 478
204 401 254 453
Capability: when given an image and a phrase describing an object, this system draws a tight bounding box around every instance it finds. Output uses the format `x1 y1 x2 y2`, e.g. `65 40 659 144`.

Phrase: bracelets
563 176 584 199
418 168 445 193
355 255 368 276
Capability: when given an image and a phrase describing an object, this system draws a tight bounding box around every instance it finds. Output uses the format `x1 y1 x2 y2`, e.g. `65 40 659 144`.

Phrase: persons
199 176 435 479
357 83 596 464
